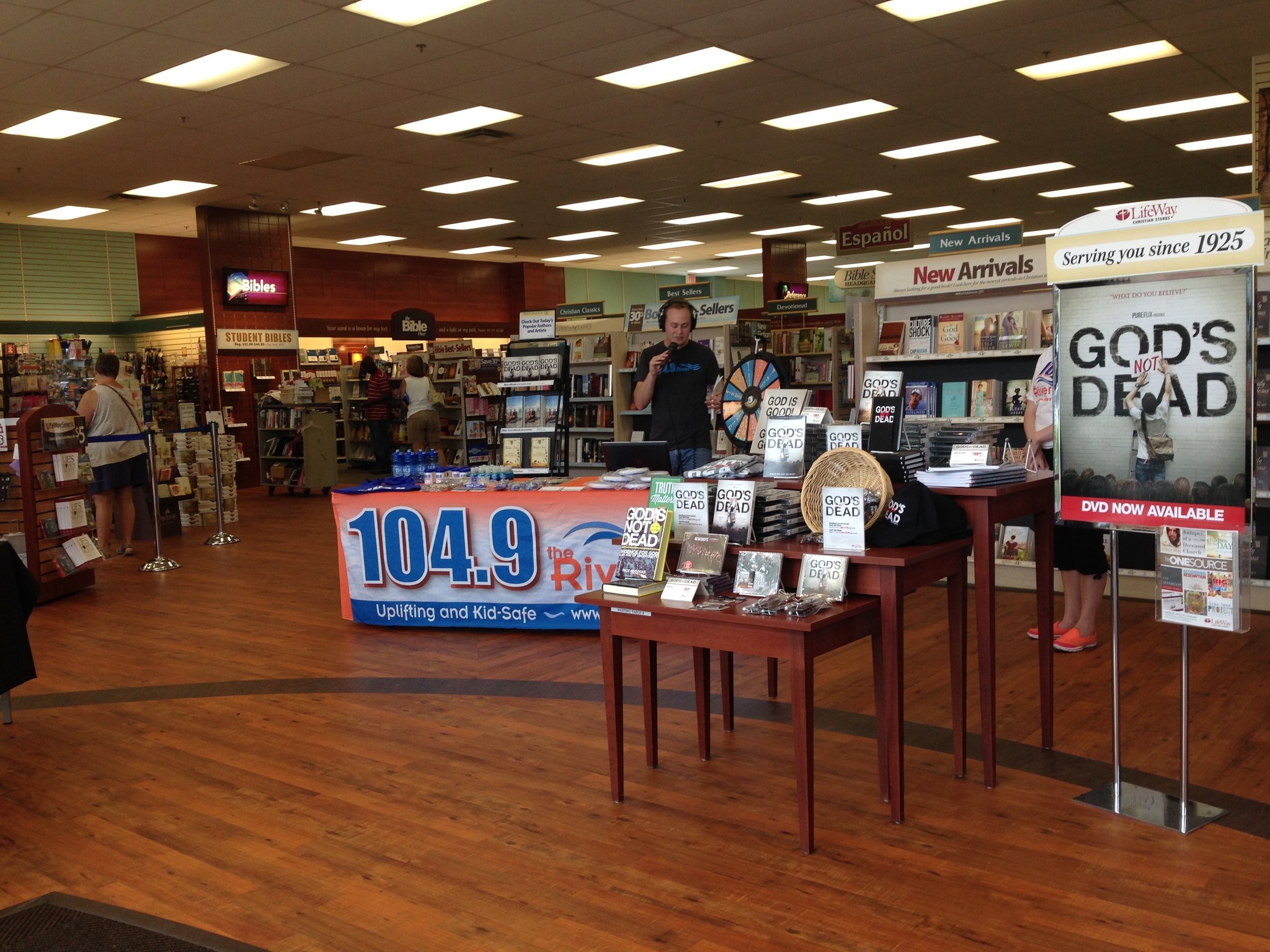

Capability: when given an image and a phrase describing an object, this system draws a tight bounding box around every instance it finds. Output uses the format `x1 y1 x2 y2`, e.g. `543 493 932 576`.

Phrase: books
602 323 904 600
34 415 110 578
856 307 1054 561
150 434 192 498
1248 291 1270 581
306 336 615 478
258 383 313 485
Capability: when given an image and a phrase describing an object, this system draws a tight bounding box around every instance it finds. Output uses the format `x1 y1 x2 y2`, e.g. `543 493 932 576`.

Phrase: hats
911 388 921 395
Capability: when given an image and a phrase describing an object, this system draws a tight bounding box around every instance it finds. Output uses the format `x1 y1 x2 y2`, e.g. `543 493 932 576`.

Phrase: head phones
656 298 698 333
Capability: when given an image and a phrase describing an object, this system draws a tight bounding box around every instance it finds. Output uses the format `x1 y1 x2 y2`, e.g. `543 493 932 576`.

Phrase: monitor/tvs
221 266 290 307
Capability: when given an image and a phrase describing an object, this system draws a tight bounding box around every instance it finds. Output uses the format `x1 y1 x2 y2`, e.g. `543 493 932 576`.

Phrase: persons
974 384 986 418
74 353 147 558
633 298 722 475
1023 345 1110 652
1007 317 1021 349
1125 357 1174 484
397 355 447 468
905 387 926 410
1060 467 1251 526
401 362 462 408
1002 311 1014 349
356 356 393 474
1160 527 1181 554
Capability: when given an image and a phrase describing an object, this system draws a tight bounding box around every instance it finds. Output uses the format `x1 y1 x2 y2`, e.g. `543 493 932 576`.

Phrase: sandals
98 548 111 558
117 545 134 555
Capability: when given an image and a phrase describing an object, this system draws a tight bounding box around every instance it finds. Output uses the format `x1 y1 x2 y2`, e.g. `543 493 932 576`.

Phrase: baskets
801 446 894 535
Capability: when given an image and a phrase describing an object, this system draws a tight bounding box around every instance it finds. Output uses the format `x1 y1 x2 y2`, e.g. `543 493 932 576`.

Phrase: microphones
660 341 679 366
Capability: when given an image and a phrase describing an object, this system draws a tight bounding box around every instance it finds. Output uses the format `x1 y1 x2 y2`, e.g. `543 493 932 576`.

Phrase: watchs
1134 383 1140 388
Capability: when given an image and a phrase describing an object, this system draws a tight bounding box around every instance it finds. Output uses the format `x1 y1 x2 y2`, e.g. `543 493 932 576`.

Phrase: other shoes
369 460 391 474
1052 628 1097 652
1027 620 1072 640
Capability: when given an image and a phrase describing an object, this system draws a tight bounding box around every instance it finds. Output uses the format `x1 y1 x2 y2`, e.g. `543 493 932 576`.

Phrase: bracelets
1164 372 1169 376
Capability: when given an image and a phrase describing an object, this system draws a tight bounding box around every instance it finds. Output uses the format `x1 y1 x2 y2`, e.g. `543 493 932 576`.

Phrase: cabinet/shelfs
257 349 500 498
490 284 1270 614
0 352 214 603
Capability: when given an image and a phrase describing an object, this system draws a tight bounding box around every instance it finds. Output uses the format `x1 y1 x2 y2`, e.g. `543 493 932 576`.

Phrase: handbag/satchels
1141 411 1174 461
428 378 445 408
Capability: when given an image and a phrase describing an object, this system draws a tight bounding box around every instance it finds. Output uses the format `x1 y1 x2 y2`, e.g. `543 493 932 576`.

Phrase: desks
575 589 880 855
612 532 972 825
331 477 650 630
685 472 1060 791
